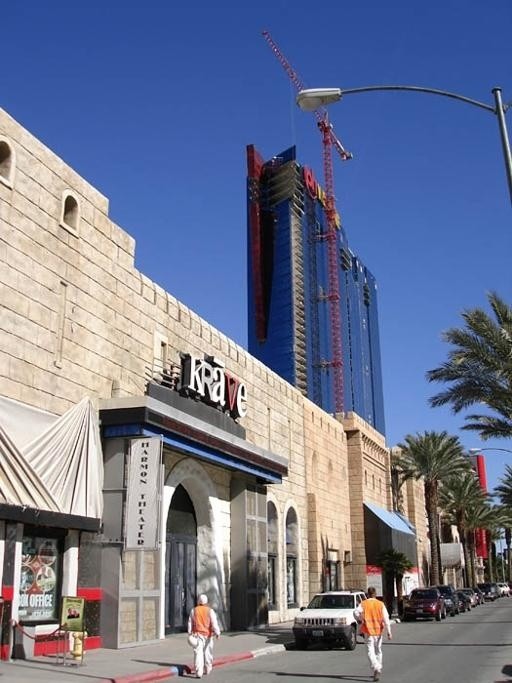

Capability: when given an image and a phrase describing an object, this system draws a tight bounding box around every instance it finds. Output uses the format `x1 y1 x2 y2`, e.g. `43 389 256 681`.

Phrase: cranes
260 28 350 409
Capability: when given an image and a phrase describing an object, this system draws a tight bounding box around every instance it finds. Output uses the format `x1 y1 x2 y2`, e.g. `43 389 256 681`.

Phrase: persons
353 587 392 681
187 594 220 679
68 608 79 618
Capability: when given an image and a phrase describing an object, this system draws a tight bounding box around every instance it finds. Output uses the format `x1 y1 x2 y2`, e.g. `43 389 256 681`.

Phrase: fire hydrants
71 631 88 661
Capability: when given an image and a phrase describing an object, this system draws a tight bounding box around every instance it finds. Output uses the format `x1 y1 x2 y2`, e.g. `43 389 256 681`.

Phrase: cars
404 582 511 621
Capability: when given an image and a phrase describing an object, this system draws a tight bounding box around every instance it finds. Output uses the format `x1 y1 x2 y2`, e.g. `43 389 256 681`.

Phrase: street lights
296 86 512 190
468 448 512 455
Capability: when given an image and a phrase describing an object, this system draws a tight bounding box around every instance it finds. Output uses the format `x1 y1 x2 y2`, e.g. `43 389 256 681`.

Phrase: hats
198 593 209 605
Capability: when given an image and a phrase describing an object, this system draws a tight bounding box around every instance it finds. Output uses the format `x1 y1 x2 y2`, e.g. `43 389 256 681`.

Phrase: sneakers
375 670 381 681
206 668 212 674
195 673 203 678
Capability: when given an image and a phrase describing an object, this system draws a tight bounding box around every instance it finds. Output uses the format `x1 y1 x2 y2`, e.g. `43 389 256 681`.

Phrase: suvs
293 590 367 650
